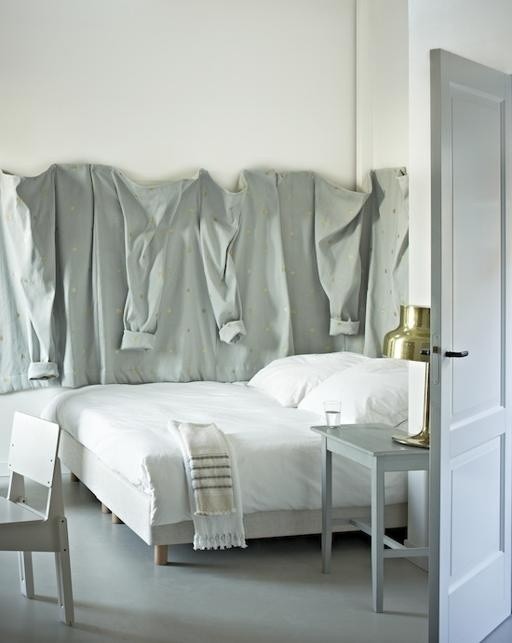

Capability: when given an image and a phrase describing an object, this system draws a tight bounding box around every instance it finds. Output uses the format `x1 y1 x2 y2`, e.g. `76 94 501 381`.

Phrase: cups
323 400 342 429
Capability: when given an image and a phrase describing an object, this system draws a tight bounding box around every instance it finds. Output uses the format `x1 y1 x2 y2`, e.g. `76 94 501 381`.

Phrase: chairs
0 413 75 626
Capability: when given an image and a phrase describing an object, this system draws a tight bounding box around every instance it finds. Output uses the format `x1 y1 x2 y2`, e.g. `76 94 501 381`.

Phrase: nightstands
403 468 429 573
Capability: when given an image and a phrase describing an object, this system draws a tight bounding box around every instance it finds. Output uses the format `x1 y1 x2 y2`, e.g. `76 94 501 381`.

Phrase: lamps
378 304 430 449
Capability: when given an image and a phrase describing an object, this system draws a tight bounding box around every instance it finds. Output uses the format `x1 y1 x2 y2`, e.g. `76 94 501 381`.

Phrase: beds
37 380 408 565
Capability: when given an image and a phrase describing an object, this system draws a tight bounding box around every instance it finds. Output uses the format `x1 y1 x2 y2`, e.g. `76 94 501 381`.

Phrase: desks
312 421 429 614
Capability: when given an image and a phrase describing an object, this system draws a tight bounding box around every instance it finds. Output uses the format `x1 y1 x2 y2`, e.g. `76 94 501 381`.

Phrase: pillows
249 349 408 429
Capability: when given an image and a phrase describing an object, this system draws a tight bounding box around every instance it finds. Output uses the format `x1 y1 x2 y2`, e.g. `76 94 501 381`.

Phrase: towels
168 418 248 551
